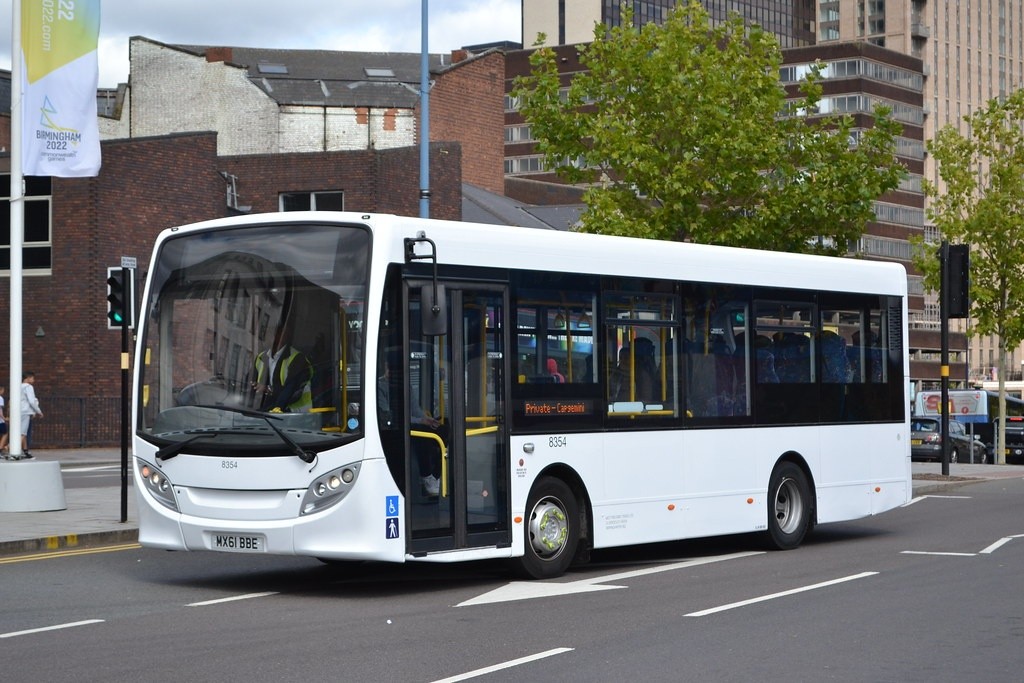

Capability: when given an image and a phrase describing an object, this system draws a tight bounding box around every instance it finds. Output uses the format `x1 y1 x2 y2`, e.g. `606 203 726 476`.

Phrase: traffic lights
106 267 137 331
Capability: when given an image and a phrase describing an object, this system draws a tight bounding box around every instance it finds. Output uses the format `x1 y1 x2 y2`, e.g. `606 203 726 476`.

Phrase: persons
485 375 502 416
232 316 313 413
0 371 43 460
379 360 450 495
547 358 565 383
612 348 652 403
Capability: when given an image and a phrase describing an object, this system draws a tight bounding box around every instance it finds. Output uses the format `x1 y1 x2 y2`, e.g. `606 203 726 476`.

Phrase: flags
20 0 102 178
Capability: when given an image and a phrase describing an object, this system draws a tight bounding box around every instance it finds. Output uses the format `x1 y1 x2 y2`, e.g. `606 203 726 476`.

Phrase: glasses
263 325 279 334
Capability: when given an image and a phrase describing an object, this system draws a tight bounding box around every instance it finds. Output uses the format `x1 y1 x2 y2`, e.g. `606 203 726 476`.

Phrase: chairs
698 328 885 415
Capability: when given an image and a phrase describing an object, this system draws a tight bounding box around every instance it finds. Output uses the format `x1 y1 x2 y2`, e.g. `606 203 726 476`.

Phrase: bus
130 210 915 579
915 385 1024 456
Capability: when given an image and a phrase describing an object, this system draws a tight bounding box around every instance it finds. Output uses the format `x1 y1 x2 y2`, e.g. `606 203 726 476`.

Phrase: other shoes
23 453 36 460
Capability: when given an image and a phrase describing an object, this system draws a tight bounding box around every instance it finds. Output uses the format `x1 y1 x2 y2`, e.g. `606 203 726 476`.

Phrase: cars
910 417 988 464
993 416 1024 459
